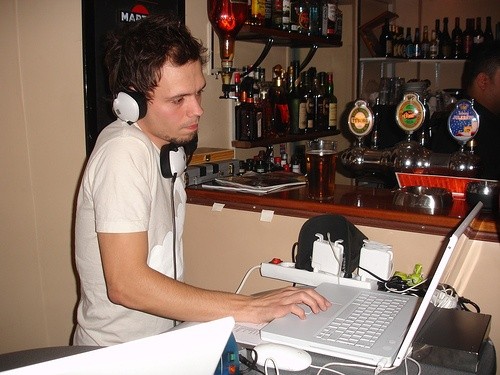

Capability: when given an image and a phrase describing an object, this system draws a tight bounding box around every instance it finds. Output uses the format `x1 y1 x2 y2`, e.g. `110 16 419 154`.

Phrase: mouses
252 345 312 371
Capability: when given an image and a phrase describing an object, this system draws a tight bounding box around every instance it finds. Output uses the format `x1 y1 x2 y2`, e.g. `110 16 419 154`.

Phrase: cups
305 139 337 200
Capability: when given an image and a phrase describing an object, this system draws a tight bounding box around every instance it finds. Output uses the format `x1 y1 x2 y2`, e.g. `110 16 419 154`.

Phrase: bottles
378 17 493 58
207 0 244 92
242 0 342 41
235 62 338 174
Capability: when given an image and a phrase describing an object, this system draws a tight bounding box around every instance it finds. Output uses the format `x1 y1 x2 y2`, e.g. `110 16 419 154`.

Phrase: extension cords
260 260 379 291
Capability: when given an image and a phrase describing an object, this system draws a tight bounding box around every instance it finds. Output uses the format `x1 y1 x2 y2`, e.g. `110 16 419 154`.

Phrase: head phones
110 86 188 178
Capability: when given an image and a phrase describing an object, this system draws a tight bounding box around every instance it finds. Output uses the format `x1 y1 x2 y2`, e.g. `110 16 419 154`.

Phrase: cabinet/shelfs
226 23 467 149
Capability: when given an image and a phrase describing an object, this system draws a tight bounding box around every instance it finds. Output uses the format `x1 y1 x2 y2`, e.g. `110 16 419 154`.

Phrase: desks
163 291 497 375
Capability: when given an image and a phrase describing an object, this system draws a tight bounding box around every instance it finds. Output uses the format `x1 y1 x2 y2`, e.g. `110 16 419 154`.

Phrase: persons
73 16 330 347
424 40 500 175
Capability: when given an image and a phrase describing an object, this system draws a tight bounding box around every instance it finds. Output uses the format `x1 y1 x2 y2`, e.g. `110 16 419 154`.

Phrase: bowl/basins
465 179 500 212
394 185 454 216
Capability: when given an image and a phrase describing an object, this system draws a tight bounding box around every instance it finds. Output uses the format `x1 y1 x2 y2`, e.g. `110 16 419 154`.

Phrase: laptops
260 202 484 368
